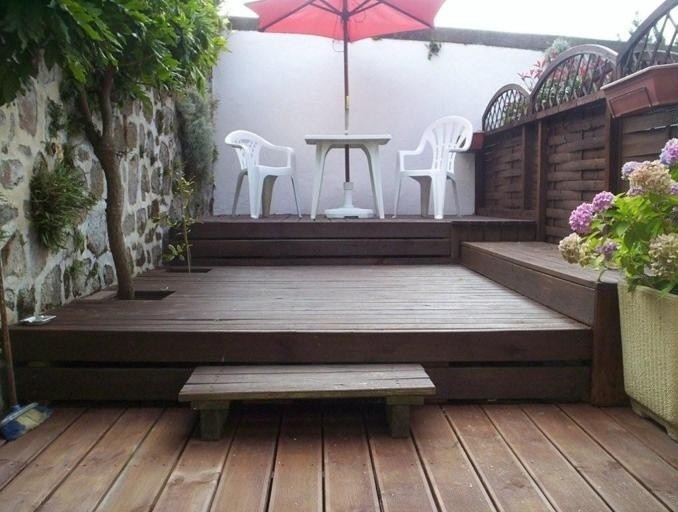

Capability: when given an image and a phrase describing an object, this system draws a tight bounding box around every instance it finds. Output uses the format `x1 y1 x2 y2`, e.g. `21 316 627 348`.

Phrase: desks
304 134 391 219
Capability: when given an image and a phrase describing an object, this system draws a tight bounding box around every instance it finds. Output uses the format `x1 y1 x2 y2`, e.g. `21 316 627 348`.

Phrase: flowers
558 138 678 299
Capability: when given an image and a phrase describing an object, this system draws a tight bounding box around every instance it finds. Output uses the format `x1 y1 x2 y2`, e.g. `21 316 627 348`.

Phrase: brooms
0 262 54 441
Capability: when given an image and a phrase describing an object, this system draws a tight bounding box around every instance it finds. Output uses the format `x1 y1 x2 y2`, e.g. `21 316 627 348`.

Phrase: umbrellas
244 0 447 182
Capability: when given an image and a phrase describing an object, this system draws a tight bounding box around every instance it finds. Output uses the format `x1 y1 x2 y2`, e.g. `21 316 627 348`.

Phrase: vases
616 280 677 441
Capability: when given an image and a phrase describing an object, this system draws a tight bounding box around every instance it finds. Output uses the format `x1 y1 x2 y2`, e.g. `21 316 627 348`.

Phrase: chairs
392 116 473 220
225 130 302 219
